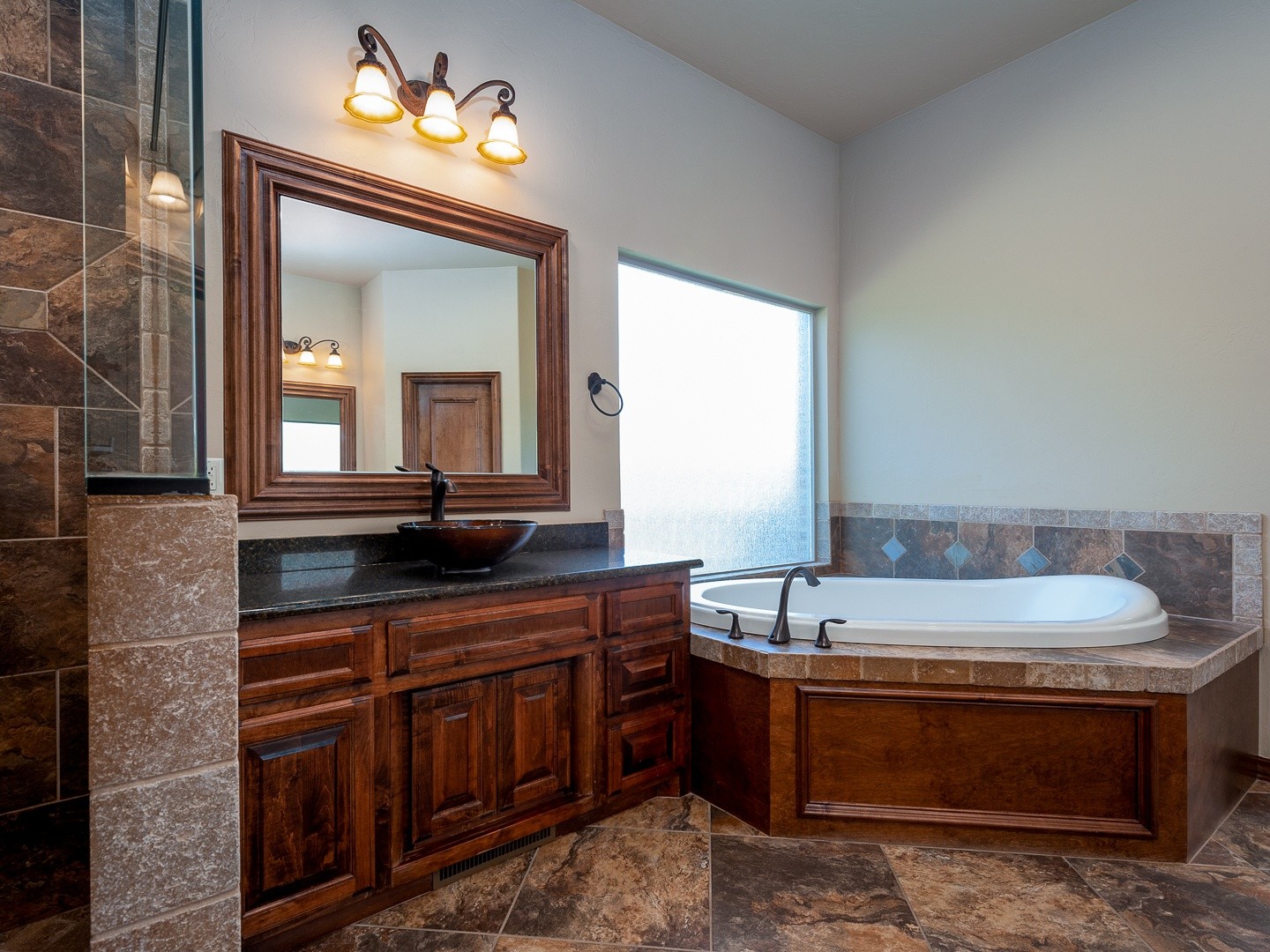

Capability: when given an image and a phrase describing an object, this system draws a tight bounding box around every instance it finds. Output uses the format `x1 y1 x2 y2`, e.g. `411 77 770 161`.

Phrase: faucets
767 565 821 644
425 463 459 521
395 466 414 472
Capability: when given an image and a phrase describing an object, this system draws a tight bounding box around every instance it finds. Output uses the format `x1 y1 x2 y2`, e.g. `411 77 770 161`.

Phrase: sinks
396 519 539 573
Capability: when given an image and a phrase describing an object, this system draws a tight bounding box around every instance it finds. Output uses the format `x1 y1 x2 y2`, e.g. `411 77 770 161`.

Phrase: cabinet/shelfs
239 571 698 939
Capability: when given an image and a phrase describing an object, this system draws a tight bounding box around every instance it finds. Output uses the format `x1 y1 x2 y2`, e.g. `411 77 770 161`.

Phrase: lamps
280 336 343 368
344 23 527 165
125 141 204 220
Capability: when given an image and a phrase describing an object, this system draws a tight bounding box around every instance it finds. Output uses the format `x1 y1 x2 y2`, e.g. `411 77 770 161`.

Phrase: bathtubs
690 574 1170 649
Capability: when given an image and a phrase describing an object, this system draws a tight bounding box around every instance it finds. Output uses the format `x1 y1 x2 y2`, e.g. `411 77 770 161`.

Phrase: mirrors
222 129 573 511
